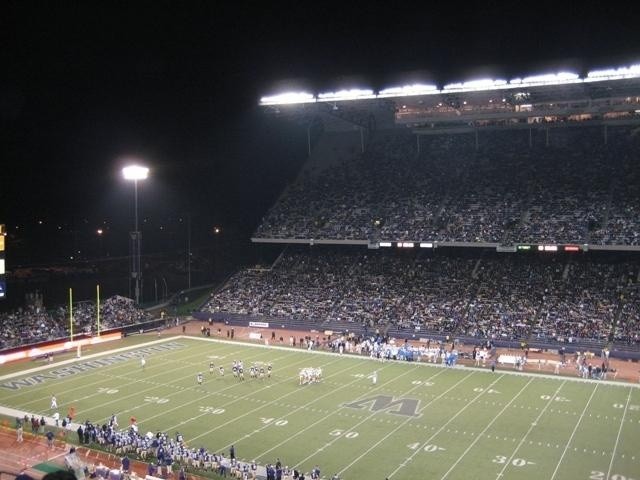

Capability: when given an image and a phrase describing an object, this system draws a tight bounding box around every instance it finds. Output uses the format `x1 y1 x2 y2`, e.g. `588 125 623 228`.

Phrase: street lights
122 166 150 308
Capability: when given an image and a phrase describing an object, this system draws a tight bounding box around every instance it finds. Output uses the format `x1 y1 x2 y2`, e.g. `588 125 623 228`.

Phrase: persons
250 362 272 379
198 375 201 383
15 394 75 448
300 366 322 385
232 360 244 380
290 334 336 351
554 346 616 380
141 355 146 368
200 109 640 345
1 294 185 350
340 330 530 373
210 362 224 375
372 371 377 385
265 457 338 479
70 415 257 480
